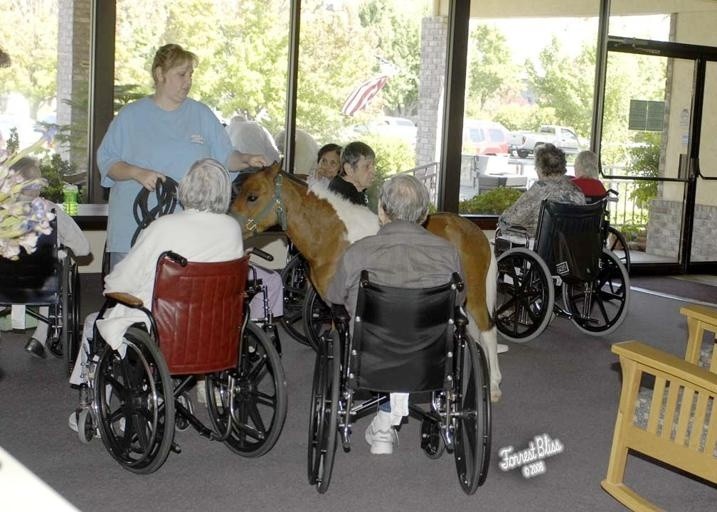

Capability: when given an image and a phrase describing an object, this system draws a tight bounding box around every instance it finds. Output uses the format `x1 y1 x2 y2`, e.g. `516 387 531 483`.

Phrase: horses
226 158 503 404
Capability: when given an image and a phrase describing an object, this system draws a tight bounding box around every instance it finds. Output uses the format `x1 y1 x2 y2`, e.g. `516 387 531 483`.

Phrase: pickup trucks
508 125 589 157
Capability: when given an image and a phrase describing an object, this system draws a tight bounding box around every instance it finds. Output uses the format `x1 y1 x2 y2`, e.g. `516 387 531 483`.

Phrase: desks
50 204 108 275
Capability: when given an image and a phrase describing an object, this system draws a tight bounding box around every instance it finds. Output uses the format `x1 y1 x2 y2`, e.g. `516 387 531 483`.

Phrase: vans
462 120 522 156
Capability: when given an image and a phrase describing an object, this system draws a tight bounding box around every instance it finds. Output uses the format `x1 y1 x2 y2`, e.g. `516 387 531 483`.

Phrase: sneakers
197 382 230 407
365 421 400 455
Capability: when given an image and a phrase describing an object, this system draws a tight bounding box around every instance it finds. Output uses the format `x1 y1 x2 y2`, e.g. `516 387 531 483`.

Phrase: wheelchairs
78 247 290 476
482 195 630 342
0 206 94 375
593 188 632 303
281 251 350 353
306 268 493 497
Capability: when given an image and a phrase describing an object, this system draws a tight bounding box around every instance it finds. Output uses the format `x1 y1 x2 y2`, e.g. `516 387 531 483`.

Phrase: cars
385 116 415 127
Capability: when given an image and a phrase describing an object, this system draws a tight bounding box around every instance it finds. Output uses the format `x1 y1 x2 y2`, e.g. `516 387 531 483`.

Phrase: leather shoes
46 329 65 359
24 338 47 361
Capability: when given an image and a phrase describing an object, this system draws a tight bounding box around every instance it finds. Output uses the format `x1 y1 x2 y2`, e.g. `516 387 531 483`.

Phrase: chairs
599 302 717 512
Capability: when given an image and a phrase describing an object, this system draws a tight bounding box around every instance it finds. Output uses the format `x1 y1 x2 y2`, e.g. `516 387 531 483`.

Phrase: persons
68 158 244 440
495 143 585 236
97 44 265 282
571 151 605 196
316 144 342 180
330 140 376 208
321 172 473 455
12 157 91 358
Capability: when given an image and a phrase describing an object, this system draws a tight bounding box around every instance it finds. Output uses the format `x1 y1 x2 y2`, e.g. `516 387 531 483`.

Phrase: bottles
60 184 79 213
